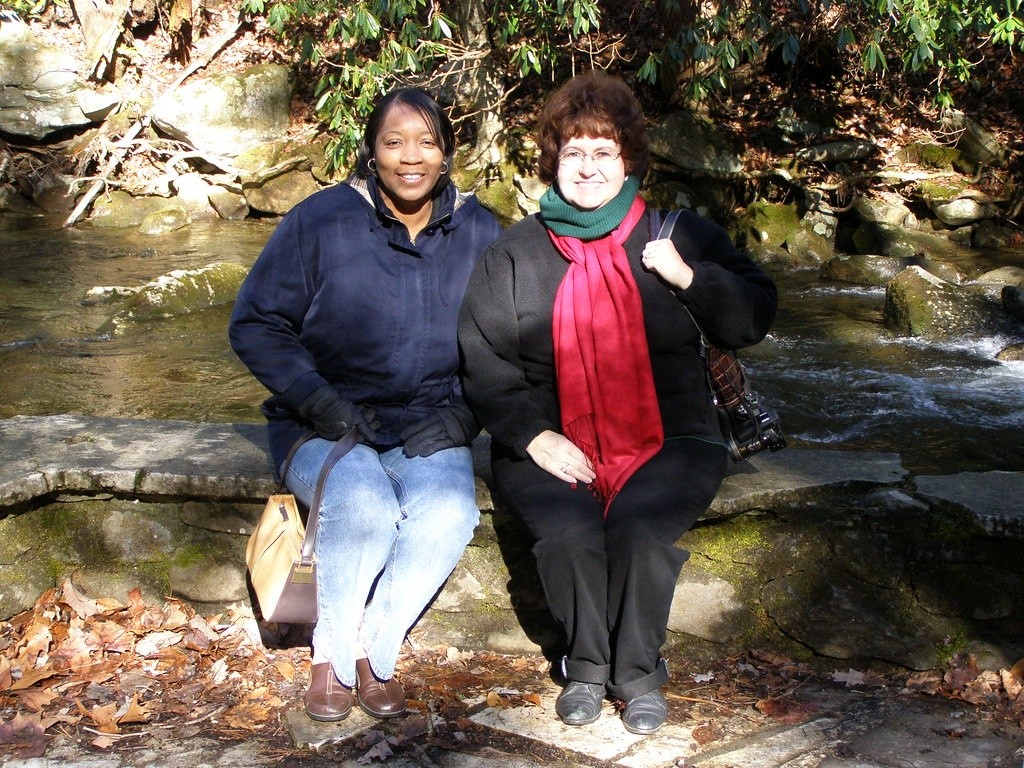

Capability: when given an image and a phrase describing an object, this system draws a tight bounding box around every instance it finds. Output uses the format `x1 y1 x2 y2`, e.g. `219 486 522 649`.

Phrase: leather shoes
622 687 668 735
556 680 607 724
304 663 352 722
356 658 406 718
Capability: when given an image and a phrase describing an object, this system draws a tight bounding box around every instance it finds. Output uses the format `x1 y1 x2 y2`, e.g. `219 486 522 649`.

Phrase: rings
560 462 570 473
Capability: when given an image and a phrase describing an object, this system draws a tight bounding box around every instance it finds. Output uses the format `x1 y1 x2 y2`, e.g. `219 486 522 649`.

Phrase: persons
457 69 778 737
228 88 507 721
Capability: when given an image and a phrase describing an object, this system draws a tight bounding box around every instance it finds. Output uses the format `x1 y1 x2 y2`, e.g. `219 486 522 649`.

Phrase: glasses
557 149 621 166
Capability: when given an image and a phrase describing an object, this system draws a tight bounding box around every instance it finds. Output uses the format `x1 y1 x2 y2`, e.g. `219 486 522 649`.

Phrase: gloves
298 383 381 443
400 403 481 457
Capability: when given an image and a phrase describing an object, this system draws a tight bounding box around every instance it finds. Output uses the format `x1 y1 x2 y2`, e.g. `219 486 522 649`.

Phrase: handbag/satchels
655 206 788 465
246 424 359 623
